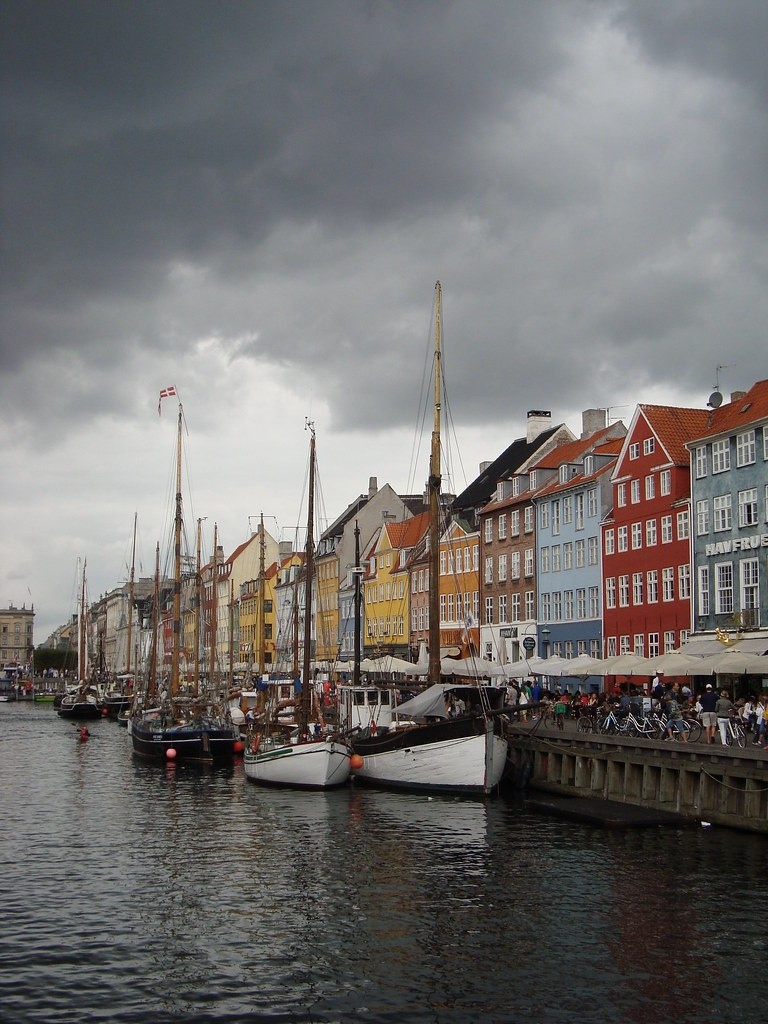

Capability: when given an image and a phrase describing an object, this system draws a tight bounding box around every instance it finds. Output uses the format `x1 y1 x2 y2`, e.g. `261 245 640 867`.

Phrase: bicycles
574 705 702 743
543 703 567 731
724 708 748 748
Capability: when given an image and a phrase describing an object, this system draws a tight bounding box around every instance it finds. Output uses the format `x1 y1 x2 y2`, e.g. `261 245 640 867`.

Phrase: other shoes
711 736 715 744
751 742 758 746
763 746 768 750
664 737 675 742
758 740 762 744
706 740 710 744
532 717 540 721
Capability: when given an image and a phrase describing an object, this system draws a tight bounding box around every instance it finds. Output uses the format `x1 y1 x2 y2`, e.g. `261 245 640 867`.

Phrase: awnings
671 639 768 656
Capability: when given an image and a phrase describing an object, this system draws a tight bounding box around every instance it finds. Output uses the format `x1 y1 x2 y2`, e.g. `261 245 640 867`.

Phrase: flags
160 386 176 397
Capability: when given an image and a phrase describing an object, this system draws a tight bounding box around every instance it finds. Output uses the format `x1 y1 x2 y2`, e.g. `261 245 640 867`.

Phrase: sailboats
51 278 515 799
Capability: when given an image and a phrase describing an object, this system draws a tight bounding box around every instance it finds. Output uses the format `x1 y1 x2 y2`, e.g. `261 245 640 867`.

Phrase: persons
0 663 768 751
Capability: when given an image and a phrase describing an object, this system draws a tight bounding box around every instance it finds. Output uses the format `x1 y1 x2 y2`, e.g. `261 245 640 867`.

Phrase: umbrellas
118 639 767 703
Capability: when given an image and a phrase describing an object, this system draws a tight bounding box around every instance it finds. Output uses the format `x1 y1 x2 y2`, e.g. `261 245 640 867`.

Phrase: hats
706 684 712 689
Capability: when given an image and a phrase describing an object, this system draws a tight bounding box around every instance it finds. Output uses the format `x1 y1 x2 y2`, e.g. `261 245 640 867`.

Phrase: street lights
541 624 552 693
